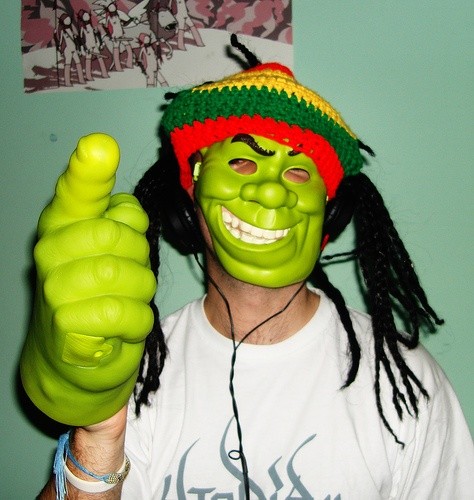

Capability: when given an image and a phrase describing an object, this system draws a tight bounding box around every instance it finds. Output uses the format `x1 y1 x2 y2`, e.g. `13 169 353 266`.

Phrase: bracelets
63 451 126 493
52 429 131 500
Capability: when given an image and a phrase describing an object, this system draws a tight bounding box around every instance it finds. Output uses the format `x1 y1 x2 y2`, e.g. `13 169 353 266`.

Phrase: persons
17 34 474 500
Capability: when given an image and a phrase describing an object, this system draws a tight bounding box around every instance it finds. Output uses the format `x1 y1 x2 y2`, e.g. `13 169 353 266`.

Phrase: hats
160 62 373 199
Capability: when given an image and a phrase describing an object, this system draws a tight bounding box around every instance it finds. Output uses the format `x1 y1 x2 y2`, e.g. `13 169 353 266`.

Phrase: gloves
20 133 159 427
